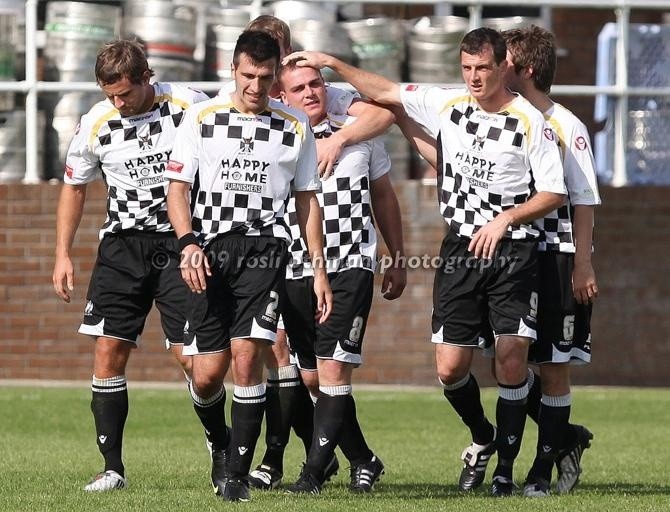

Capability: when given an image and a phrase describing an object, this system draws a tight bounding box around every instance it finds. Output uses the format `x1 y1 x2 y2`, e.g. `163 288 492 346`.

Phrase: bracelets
179 233 199 252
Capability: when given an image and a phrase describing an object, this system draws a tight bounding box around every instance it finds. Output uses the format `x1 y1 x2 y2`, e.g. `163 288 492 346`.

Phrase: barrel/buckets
0 0 545 184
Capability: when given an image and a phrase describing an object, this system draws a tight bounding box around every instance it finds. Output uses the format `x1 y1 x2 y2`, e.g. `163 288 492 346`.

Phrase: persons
395 23 603 499
216 15 396 490
280 28 569 499
52 36 211 492
161 31 334 501
280 56 408 495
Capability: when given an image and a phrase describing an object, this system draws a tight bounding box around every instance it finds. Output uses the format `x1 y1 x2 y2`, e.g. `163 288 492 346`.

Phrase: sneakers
459 425 498 490
247 462 283 490
286 452 339 497
491 474 520 497
522 476 552 498
350 455 385 495
205 427 249 503
553 424 593 496
84 470 128 492
